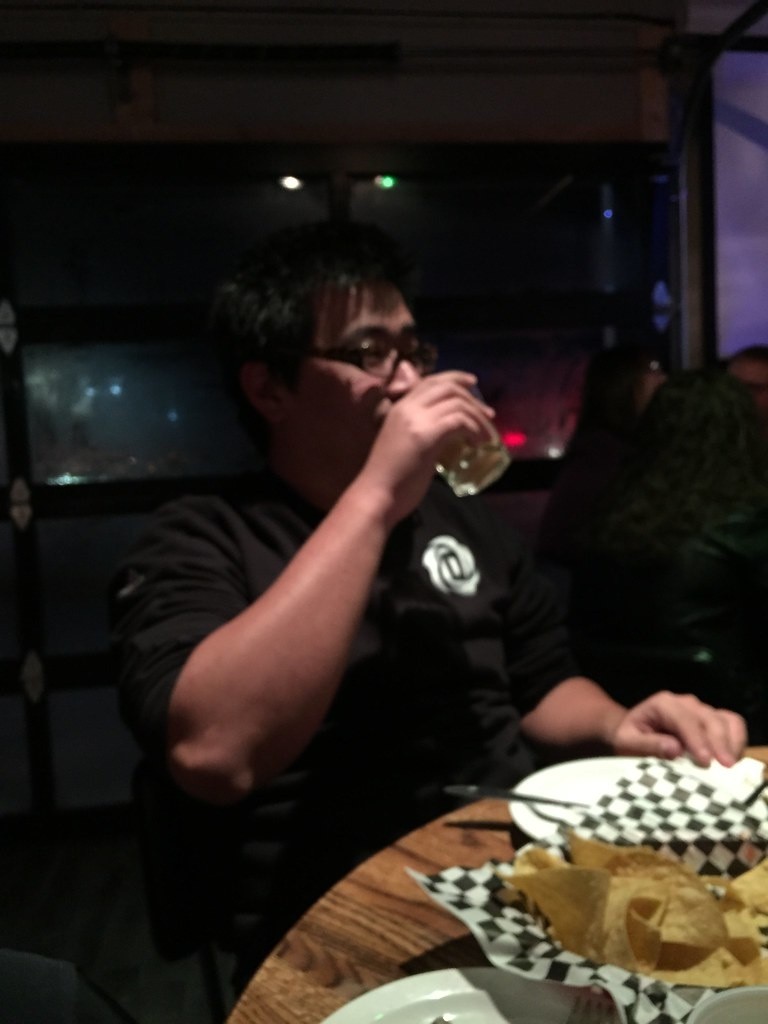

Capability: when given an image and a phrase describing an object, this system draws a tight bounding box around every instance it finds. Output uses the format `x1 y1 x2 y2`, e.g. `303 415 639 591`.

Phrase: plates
509 753 768 846
313 965 678 1024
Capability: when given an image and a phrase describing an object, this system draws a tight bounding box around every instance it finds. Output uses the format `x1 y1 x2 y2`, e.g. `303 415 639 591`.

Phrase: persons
533 342 768 770
105 219 748 999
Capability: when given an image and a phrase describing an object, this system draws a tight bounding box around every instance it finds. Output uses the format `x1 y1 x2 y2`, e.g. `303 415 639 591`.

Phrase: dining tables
229 744 768 1023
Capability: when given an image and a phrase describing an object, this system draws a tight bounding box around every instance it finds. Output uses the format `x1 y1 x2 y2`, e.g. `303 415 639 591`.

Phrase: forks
564 996 616 1024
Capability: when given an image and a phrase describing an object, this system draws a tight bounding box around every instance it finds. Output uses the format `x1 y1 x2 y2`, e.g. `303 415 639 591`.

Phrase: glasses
282 333 439 379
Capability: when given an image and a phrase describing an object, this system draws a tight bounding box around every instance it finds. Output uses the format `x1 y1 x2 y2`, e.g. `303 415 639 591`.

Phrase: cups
432 384 510 496
686 985 768 1024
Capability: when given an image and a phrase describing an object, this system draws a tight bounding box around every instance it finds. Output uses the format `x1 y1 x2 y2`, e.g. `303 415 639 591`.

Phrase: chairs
132 755 309 1019
